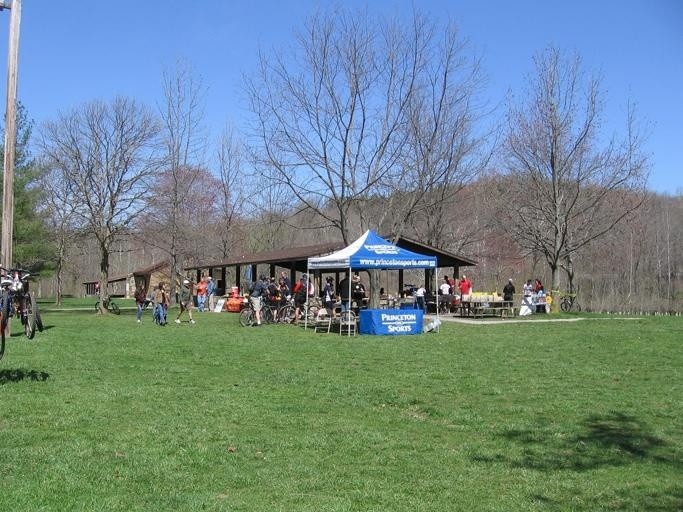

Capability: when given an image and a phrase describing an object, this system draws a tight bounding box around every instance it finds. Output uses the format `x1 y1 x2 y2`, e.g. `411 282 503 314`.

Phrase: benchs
458 306 516 320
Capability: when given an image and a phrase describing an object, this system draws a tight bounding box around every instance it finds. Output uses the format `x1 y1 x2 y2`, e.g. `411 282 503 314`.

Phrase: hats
351 276 360 283
183 280 190 283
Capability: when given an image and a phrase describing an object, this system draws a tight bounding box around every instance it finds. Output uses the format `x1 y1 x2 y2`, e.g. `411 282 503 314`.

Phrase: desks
459 301 516 319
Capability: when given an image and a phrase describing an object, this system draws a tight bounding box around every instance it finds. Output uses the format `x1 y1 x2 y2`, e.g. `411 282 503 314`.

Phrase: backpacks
247 280 262 291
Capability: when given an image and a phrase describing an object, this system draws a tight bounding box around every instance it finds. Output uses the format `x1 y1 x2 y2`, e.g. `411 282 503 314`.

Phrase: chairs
314 308 356 336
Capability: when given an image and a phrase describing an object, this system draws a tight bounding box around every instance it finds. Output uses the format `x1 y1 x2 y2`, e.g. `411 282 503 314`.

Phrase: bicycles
94 294 120 315
238 297 356 326
558 294 581 313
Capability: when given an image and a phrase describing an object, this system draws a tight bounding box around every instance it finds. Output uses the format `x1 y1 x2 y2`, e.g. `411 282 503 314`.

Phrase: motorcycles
0 263 43 339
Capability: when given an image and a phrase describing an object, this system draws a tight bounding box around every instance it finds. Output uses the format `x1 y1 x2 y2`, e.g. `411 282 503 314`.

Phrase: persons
521 279 532 295
133 282 146 324
502 278 515 316
205 276 216 313
155 281 170 327
149 285 160 323
173 279 196 325
244 271 473 328
533 279 544 294
194 278 207 313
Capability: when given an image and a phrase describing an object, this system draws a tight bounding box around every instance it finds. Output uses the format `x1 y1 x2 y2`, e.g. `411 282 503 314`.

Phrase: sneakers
174 318 180 324
189 320 195 324
